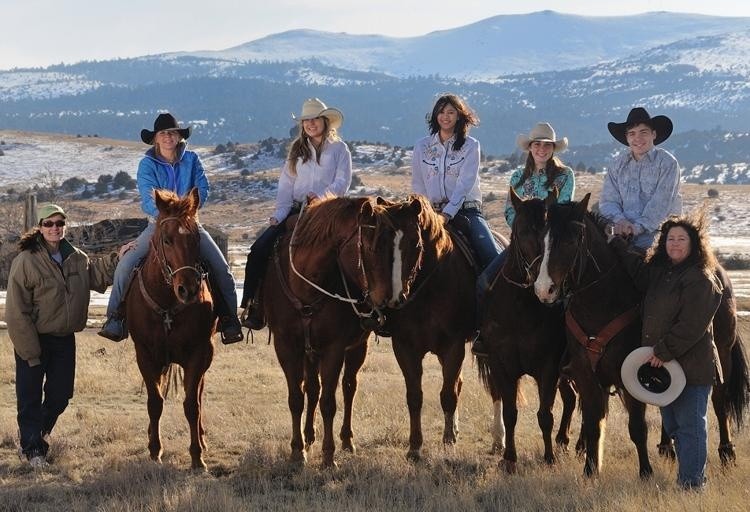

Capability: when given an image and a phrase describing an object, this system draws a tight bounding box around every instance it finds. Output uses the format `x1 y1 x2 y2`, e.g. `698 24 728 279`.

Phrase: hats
141 113 192 145
38 204 69 224
622 347 686 406
516 123 568 153
608 107 674 147
291 98 343 130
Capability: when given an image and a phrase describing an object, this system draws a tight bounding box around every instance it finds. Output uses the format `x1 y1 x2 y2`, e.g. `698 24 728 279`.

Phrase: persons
98 113 242 344
609 222 722 490
242 97 352 332
6 205 135 467
594 107 681 291
505 124 575 230
410 96 501 268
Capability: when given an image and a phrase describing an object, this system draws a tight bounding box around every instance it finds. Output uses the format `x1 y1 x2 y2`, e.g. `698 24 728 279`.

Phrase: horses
260 197 391 471
377 196 510 462
122 187 219 475
535 192 750 484
492 185 565 472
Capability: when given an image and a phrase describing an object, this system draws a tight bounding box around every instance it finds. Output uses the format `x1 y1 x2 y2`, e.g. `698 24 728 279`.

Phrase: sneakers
29 456 49 466
243 303 265 330
472 335 491 357
220 315 242 340
103 316 129 339
17 429 27 462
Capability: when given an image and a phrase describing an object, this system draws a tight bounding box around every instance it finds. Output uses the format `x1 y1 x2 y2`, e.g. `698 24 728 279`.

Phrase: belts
434 201 477 211
147 213 200 224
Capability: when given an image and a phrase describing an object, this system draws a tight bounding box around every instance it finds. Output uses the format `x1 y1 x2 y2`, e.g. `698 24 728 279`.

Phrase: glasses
42 220 65 227
625 130 652 137
